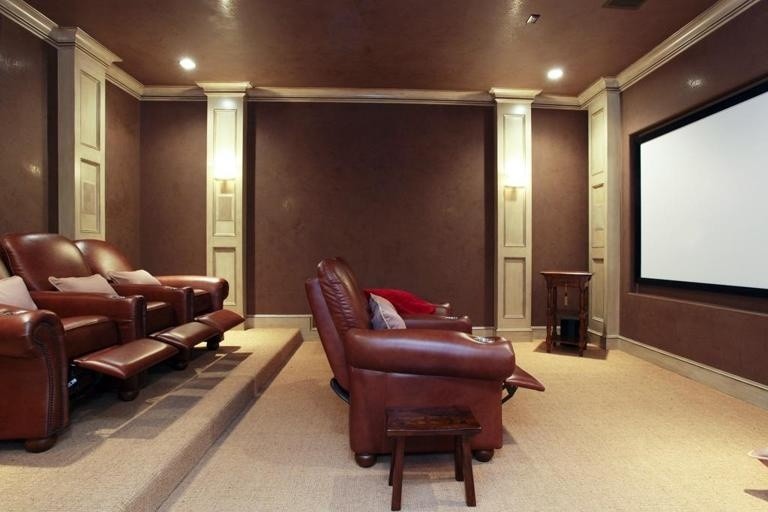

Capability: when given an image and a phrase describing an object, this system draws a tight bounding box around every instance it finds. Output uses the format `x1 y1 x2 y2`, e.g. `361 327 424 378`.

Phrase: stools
383 404 481 511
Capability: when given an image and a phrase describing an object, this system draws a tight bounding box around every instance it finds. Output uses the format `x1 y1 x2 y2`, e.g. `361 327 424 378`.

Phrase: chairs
73 238 245 351
2 229 219 390
0 249 179 453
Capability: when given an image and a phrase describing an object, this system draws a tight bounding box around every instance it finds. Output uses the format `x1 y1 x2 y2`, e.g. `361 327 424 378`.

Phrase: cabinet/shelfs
540 269 594 355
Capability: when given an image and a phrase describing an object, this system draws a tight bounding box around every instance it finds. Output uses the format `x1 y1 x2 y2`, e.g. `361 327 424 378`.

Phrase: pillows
0 275 38 312
47 274 121 300
107 267 162 285
